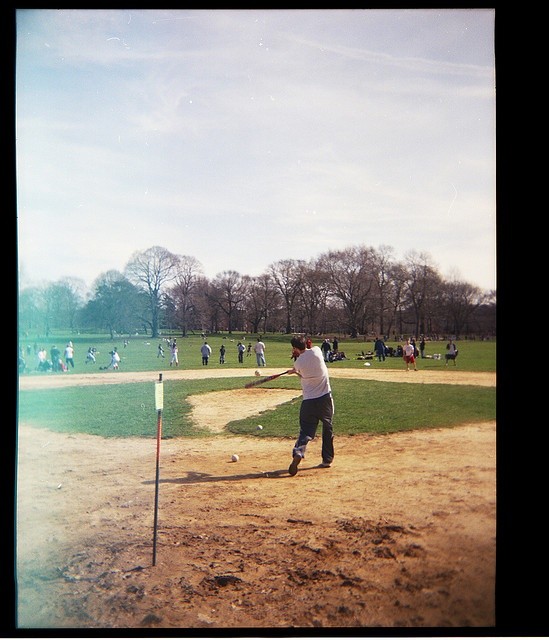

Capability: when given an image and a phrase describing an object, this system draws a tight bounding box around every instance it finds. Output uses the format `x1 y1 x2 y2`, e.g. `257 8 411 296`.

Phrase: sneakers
289 453 302 476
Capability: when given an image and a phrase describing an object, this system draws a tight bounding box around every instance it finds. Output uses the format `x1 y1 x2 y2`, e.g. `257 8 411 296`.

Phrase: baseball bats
245 371 288 389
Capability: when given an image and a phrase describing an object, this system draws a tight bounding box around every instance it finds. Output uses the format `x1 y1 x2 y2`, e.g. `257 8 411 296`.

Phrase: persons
157 344 165 358
219 345 226 364
27 344 31 355
174 338 177 343
445 339 458 366
85 346 97 364
254 338 266 367
332 337 338 352
402 337 418 371
170 338 173 352
246 343 252 358
37 347 51 371
34 343 38 355
50 345 61 372
114 347 117 352
419 336 425 359
170 343 179 367
237 342 245 363
108 350 120 369
69 339 73 347
374 337 386 361
321 340 331 363
287 335 334 476
306 337 312 348
63 345 75 368
201 342 212 366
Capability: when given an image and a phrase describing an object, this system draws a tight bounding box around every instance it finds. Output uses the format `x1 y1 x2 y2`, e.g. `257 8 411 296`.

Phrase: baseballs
231 454 239 462
364 362 370 367
256 425 263 430
255 370 261 377
